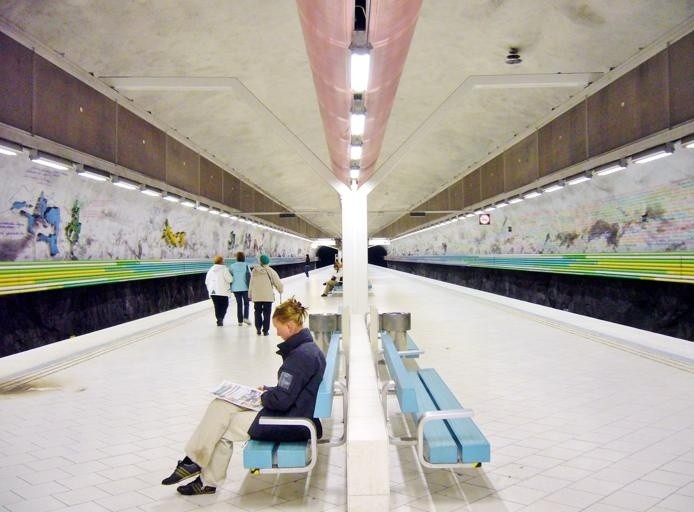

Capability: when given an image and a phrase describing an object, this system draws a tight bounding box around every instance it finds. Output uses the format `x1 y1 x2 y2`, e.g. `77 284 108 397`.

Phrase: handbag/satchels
246 272 250 287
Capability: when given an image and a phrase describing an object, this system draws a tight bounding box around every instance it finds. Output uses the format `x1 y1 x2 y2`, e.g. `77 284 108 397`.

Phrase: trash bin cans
379 313 411 364
309 313 341 359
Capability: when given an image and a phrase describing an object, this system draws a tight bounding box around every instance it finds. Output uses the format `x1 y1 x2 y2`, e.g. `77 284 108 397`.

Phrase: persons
204 255 234 327
304 253 343 297
247 254 284 336
161 294 326 496
229 251 252 326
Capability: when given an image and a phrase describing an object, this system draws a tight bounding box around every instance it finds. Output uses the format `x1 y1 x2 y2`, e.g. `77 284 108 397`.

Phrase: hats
260 255 270 264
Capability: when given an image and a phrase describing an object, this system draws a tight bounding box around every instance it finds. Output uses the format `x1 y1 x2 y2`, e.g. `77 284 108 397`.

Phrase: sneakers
177 476 215 495
162 463 201 484
238 319 269 337
217 318 223 325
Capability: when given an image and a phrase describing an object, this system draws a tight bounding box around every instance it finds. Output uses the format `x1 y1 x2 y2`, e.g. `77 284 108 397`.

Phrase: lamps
349 1 368 192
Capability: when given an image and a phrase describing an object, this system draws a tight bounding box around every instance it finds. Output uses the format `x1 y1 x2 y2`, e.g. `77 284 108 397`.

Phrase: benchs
242 331 491 477
331 272 343 296
